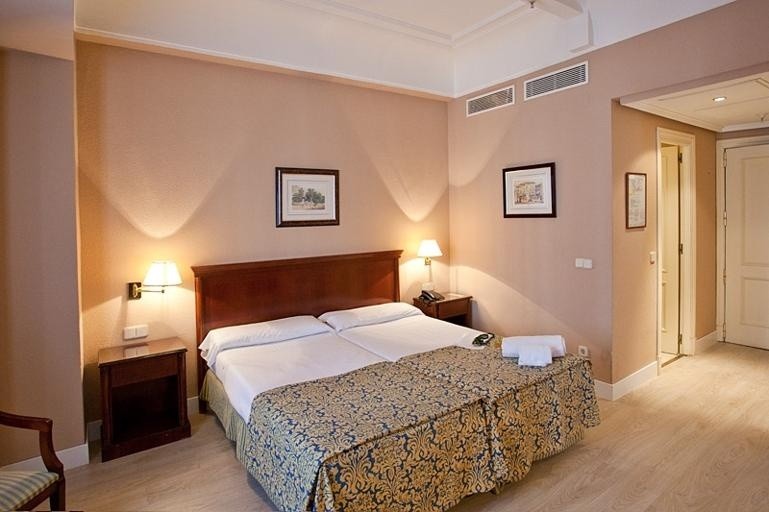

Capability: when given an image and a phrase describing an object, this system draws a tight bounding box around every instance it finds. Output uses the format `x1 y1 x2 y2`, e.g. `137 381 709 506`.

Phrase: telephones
420 289 445 302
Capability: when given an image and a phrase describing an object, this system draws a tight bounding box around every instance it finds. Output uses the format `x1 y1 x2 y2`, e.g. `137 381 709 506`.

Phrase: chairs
0 408 66 512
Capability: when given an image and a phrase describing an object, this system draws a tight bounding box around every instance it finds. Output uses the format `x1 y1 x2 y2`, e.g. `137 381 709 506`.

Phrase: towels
501 336 567 366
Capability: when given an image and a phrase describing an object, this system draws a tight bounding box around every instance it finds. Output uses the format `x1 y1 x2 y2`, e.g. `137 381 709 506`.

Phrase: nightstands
97 336 191 463
413 292 472 326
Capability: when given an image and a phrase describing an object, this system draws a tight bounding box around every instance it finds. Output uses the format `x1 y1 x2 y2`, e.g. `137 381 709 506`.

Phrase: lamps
129 251 182 298
418 239 443 287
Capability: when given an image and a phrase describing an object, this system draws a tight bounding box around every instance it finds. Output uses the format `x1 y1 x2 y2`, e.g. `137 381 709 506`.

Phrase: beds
191 248 599 512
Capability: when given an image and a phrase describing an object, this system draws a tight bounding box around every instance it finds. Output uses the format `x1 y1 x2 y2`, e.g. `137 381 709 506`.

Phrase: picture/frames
275 167 339 226
626 171 647 230
502 161 556 218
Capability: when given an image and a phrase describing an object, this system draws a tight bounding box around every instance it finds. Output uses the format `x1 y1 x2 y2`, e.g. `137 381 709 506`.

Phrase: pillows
320 301 418 331
207 314 332 350
